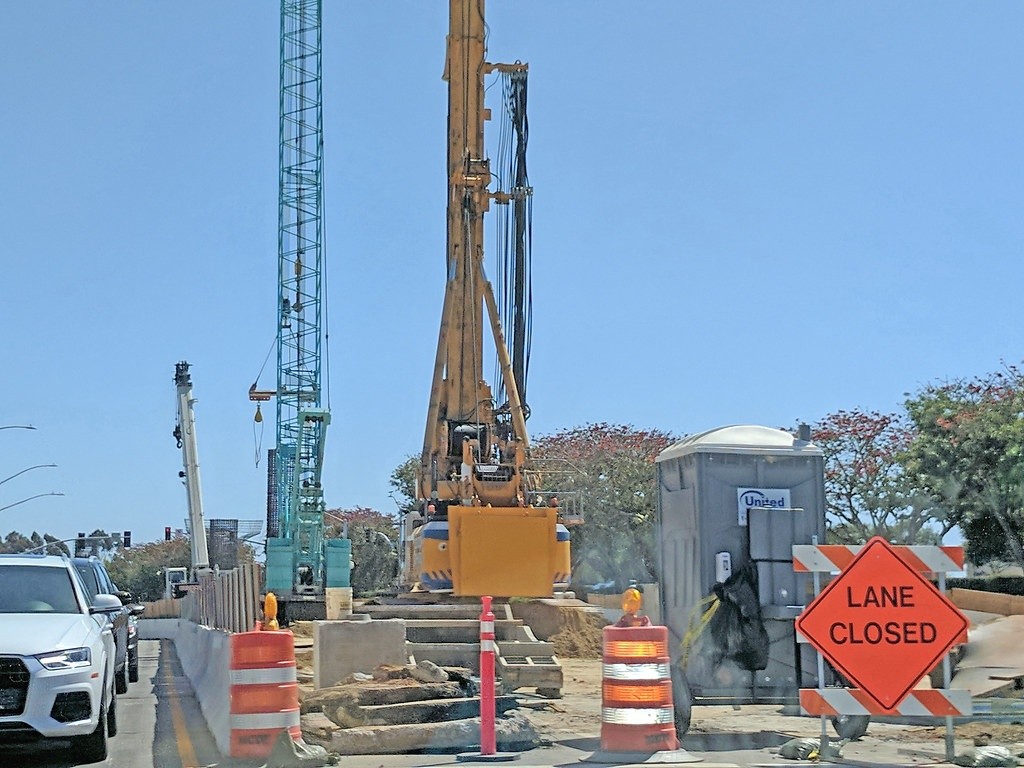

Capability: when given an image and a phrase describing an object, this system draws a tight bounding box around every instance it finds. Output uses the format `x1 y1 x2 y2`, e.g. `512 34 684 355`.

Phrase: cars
0 556 145 763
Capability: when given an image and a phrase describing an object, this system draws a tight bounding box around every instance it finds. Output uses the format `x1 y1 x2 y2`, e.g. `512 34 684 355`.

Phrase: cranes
246 1 355 605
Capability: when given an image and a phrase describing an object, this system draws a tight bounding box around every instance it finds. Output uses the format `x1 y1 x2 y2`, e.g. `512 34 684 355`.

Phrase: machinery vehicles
399 0 589 598
161 360 210 597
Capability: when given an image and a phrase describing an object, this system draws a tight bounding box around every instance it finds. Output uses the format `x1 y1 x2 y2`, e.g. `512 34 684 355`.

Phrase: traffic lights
166 527 171 541
